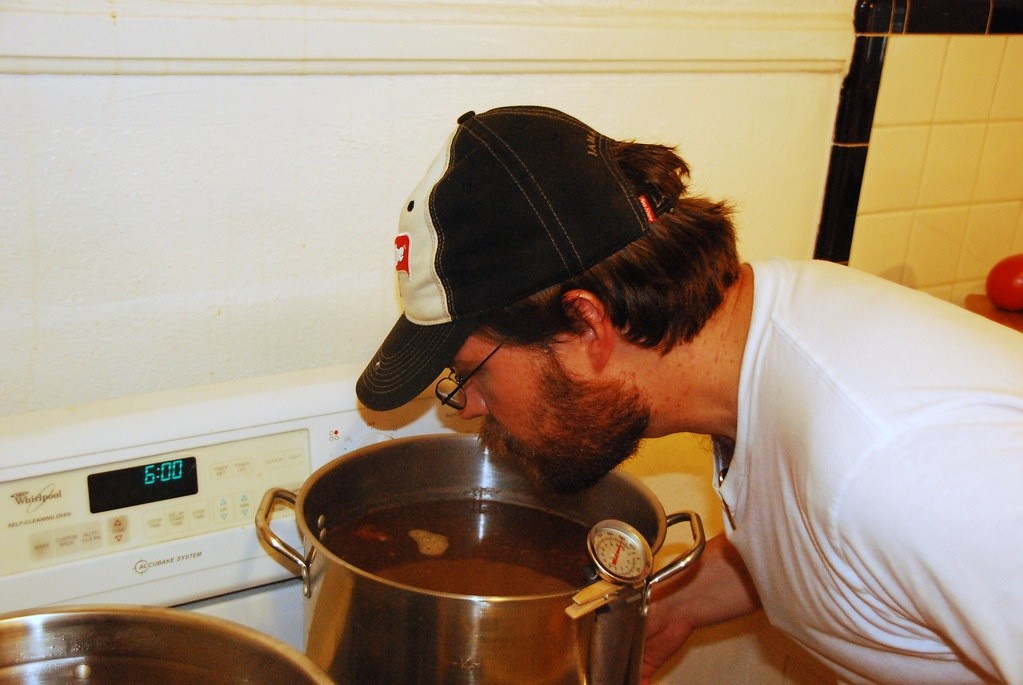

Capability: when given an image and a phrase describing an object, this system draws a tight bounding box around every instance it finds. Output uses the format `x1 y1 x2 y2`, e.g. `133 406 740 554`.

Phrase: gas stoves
1 381 484 655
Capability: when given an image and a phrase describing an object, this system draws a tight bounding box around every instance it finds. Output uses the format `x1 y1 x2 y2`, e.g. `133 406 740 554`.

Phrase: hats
356 103 680 413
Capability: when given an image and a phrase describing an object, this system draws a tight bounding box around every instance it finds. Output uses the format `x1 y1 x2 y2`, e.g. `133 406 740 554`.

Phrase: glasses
435 307 559 412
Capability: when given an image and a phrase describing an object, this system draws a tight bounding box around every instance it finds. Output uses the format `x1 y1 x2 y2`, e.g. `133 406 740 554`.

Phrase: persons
356 106 1023 685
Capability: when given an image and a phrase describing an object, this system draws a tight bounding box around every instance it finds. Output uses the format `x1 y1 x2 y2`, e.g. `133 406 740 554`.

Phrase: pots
255 430 707 685
0 603 336 685
